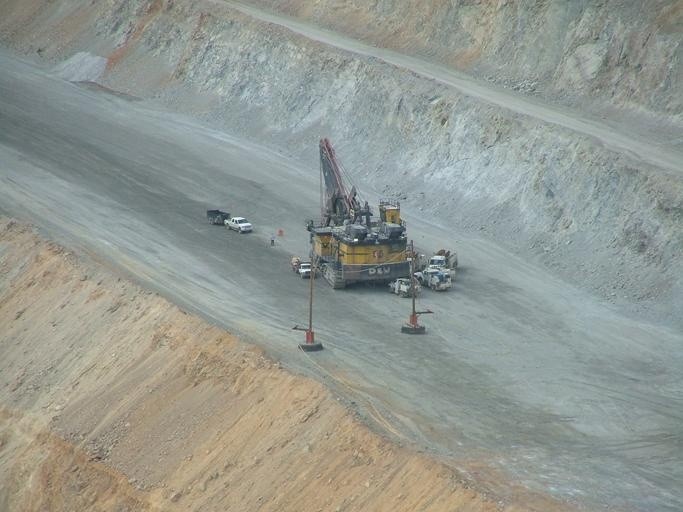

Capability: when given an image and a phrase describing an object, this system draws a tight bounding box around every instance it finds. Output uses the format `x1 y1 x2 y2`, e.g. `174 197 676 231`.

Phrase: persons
270 231 275 247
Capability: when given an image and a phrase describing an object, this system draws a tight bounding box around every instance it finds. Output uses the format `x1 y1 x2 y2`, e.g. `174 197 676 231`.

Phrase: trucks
208 209 230 225
424 269 452 291
291 258 316 278
394 278 422 298
428 250 457 270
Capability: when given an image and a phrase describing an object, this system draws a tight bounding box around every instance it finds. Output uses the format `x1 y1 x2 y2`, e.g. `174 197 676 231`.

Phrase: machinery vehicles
304 138 410 290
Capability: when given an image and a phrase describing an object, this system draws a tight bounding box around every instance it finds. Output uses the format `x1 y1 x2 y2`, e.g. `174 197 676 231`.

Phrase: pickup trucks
224 217 253 233
